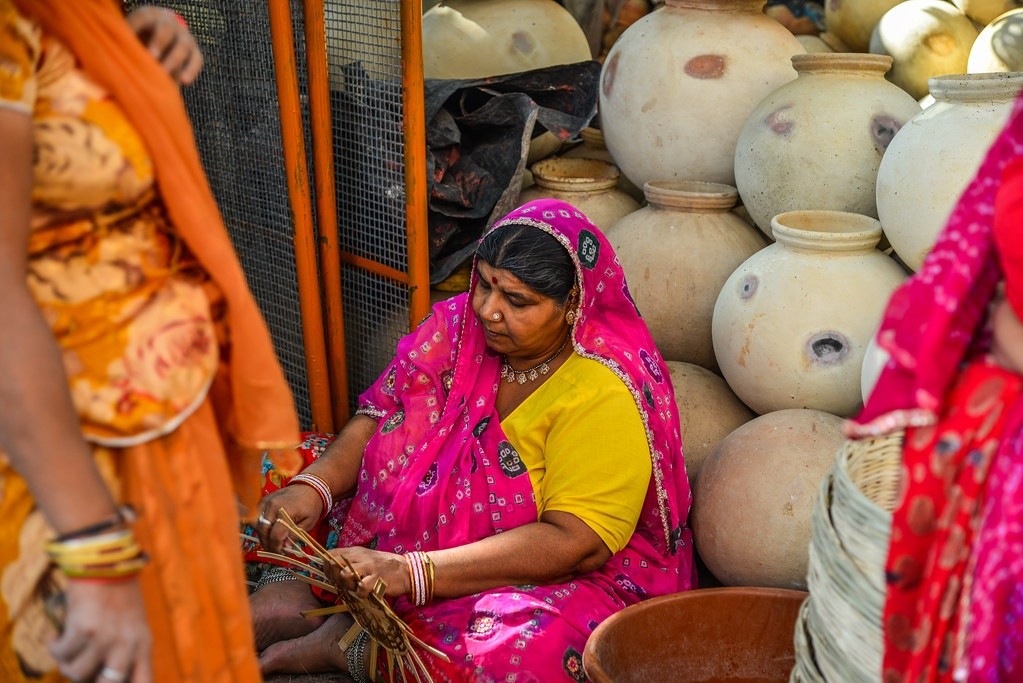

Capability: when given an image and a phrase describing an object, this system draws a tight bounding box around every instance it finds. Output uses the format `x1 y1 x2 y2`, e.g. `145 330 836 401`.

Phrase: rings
258 512 271 526
99 666 127 683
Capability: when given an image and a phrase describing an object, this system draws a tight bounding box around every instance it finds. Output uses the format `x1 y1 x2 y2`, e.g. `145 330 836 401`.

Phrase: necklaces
501 335 571 385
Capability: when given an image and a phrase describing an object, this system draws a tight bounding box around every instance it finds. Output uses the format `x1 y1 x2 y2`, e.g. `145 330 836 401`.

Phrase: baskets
786 429 906 683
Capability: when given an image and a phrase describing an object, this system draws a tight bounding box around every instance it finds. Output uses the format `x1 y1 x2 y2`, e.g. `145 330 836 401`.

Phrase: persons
245 199 691 683
844 87 1022 683
0 0 303 683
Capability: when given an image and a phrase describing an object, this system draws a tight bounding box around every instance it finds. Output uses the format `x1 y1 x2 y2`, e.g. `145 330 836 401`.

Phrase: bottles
422 0 1023 586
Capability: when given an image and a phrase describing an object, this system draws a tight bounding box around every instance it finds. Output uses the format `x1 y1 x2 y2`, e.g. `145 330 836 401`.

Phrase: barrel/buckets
580 587 811 683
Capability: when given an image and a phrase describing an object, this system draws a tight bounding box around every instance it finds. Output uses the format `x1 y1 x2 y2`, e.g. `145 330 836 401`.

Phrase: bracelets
403 551 435 607
40 502 149 585
285 472 334 519
